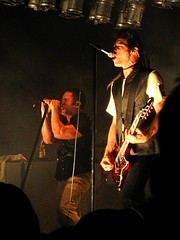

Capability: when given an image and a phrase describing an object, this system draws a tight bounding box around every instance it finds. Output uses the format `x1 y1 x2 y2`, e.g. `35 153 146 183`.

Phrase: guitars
108 96 154 192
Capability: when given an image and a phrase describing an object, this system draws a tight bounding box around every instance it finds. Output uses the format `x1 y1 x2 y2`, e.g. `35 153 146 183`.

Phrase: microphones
90 43 115 58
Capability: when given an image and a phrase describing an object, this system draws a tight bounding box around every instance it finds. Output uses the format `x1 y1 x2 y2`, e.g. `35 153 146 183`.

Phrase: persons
100 32 166 190
41 90 92 222
0 182 40 240
66 82 180 240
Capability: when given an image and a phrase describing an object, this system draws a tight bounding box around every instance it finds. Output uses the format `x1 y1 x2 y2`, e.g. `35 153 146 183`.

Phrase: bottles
39 139 47 159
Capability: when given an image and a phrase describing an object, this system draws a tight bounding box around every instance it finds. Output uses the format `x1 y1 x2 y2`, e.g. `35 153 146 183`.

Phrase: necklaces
122 63 135 70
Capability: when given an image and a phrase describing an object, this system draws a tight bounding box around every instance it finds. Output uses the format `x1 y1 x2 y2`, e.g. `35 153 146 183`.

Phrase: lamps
88 0 114 23
59 0 84 20
151 0 180 8
115 0 145 29
27 0 58 12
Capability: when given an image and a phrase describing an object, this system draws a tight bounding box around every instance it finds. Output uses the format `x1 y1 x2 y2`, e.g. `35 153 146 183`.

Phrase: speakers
4 158 104 234
